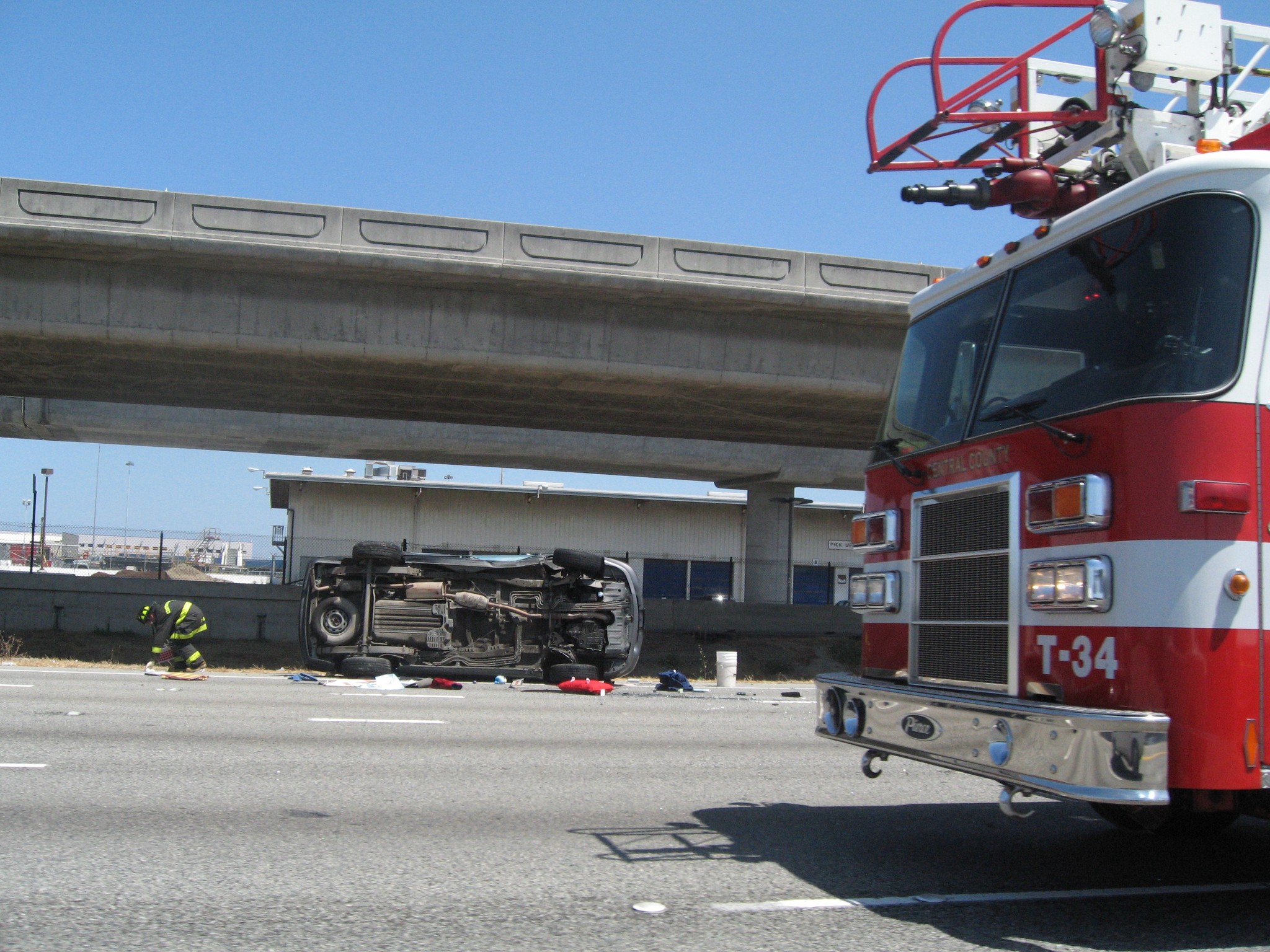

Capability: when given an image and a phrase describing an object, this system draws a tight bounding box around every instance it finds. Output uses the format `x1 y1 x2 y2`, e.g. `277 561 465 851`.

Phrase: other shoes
185 661 207 673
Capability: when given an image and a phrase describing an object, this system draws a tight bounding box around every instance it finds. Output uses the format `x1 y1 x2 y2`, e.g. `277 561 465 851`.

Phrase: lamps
248 468 266 479
253 486 270 495
537 484 548 498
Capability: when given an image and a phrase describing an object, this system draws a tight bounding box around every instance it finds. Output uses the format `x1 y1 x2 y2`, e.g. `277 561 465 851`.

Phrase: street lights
21 499 32 566
35 468 54 573
122 461 135 557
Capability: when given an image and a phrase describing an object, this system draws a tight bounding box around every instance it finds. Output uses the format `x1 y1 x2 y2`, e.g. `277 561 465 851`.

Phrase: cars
293 536 649 684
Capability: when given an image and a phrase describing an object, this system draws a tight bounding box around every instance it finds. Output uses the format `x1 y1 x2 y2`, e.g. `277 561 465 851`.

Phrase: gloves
145 661 156 670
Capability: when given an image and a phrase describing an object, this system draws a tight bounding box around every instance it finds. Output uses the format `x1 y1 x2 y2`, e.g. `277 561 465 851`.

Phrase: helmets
135 605 152 624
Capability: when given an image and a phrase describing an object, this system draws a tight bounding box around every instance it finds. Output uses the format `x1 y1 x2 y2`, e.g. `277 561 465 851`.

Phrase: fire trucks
806 1 1270 850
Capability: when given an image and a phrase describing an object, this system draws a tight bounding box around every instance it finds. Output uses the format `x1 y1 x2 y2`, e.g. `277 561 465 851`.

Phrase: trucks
72 555 107 570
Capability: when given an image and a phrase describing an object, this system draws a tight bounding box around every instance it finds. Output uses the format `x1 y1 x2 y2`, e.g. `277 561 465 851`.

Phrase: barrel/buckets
716 651 738 687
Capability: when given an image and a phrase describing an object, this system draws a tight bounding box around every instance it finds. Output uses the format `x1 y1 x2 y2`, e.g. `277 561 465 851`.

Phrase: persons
136 600 208 673
